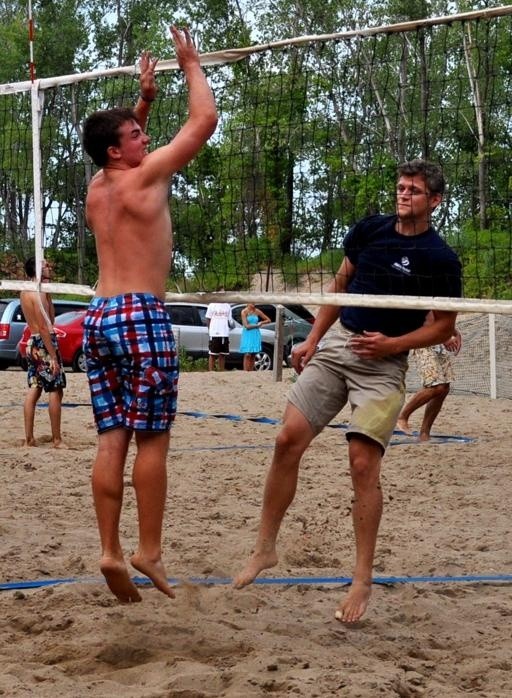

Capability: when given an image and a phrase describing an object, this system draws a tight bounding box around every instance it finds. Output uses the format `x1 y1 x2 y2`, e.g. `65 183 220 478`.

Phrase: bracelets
141 94 156 102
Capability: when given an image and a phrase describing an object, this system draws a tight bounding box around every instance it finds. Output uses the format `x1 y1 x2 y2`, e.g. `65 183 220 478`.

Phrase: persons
230 157 461 626
241 302 271 371
17 255 71 450
205 286 233 372
78 26 219 604
397 314 461 443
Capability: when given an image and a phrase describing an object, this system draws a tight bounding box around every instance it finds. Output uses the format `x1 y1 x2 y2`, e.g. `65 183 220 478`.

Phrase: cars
19 309 89 371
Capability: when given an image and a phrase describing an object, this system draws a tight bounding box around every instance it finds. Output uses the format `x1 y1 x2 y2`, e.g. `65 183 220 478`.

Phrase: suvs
227 301 315 365
164 302 274 372
1 298 89 371
